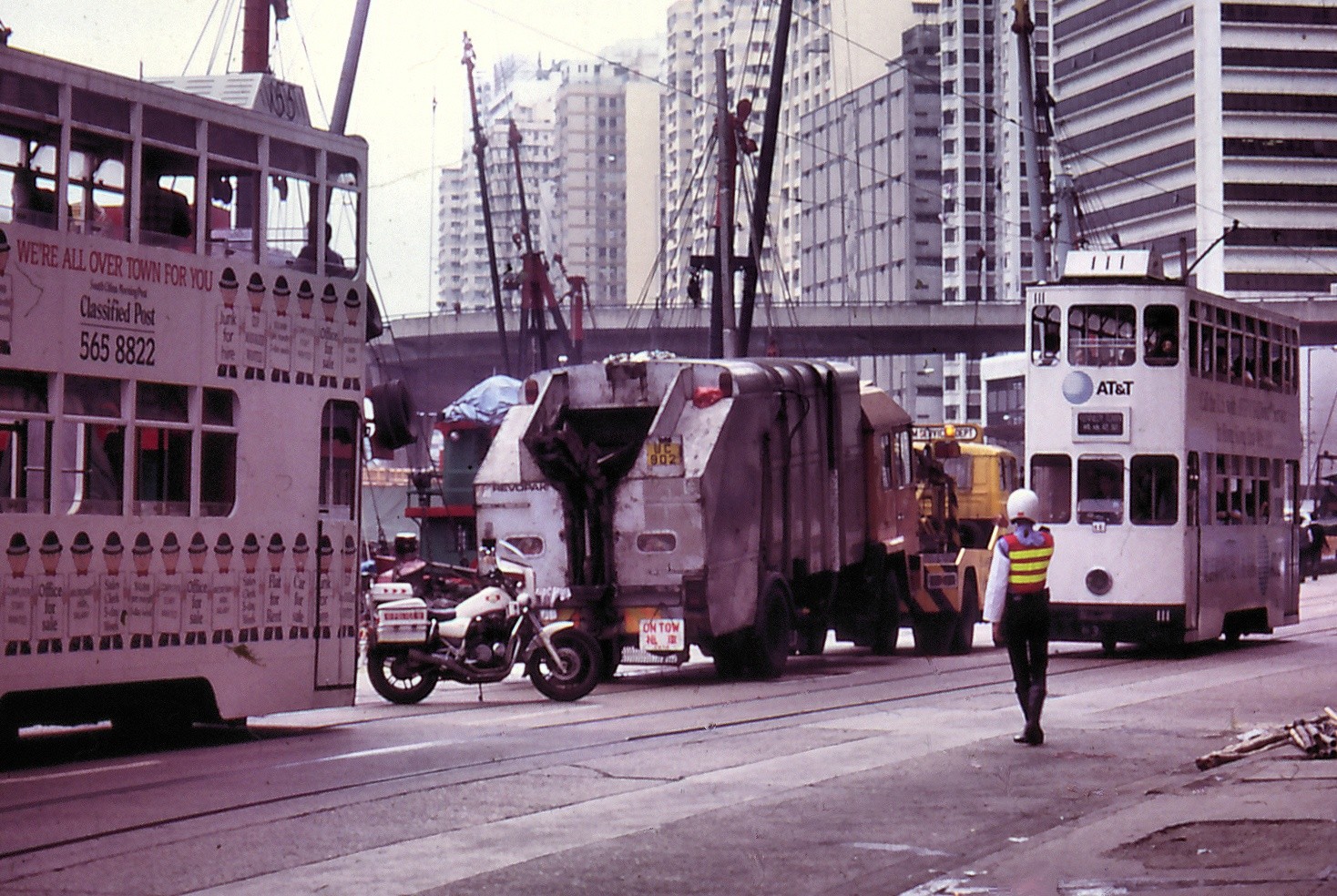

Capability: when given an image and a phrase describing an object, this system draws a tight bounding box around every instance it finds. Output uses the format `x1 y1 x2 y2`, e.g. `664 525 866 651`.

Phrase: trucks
477 349 1026 684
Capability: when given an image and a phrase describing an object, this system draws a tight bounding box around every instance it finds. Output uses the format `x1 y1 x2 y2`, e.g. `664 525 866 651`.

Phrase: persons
1298 512 1330 582
1149 339 1177 360
292 219 346 278
982 488 1055 749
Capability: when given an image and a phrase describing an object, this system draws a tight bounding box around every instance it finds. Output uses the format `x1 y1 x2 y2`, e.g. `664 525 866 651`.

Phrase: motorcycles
359 538 605 701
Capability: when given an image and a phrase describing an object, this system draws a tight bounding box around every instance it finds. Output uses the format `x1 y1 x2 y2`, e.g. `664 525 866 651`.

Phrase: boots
1013 685 1047 745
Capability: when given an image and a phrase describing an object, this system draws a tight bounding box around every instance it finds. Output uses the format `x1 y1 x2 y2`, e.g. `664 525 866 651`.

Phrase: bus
1021 226 1318 657
0 29 378 748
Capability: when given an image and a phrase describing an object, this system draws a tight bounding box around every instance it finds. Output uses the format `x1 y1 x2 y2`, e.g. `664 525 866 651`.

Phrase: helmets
1006 487 1039 522
